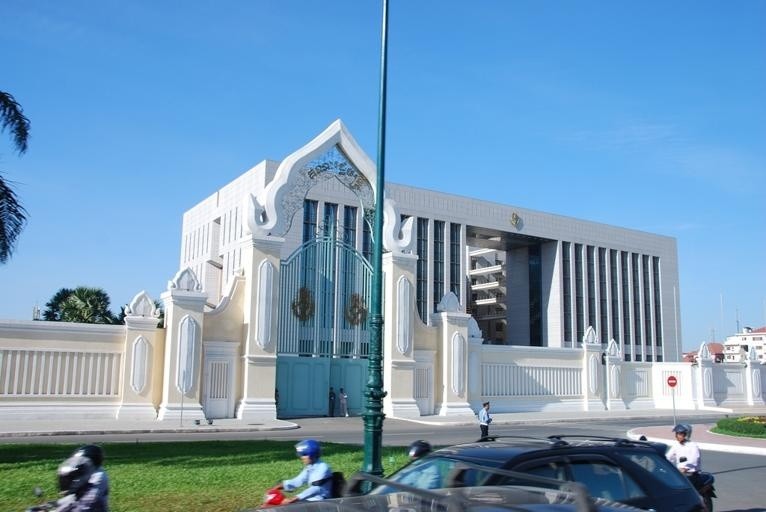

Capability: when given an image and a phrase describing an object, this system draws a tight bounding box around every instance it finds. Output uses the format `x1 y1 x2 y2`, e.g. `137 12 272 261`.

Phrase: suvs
241 462 661 511
369 431 711 511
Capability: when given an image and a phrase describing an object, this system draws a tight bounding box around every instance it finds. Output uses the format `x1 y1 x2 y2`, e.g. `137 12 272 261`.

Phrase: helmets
58 446 101 490
408 440 432 457
672 424 692 441
295 441 321 463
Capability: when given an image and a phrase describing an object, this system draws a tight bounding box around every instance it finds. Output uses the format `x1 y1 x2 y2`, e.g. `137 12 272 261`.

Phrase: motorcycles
258 478 300 507
687 469 720 512
23 485 66 511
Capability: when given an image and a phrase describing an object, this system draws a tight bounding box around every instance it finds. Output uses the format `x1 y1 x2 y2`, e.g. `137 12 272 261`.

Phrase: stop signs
668 375 678 388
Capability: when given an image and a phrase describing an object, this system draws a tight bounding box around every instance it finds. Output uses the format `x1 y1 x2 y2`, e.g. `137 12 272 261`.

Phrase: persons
272 440 332 505
329 388 336 417
39 457 107 512
339 388 349 417
479 402 492 441
665 423 702 486
275 389 281 419
77 445 109 499
400 439 441 490
